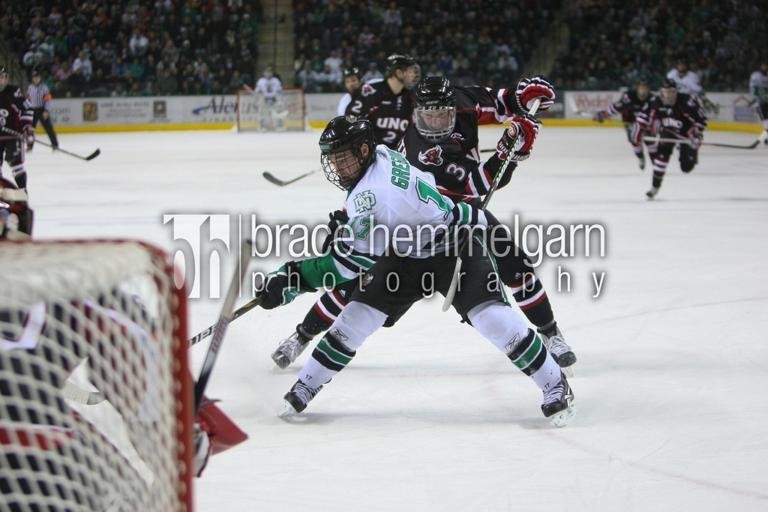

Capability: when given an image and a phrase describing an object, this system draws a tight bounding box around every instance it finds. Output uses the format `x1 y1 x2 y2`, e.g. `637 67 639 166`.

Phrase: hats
180 27 187 33
482 32 489 37
32 71 40 77
600 34 607 39
698 58 710 66
703 32 711 37
30 44 36 49
500 45 509 55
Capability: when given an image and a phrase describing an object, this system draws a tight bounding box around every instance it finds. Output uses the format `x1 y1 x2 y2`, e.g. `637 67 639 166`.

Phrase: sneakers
27 144 33 150
271 333 310 369
536 321 577 368
652 160 655 165
541 369 574 417
52 145 58 149
639 157 645 169
646 186 660 198
284 378 332 413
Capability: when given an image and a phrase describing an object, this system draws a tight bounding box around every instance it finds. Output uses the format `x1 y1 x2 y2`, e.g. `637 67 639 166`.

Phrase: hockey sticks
642 128 768 150
243 83 288 118
0 125 101 161
441 100 542 312
263 168 322 186
62 293 265 406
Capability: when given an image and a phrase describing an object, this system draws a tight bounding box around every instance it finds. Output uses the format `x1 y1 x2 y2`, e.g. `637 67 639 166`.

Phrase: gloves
594 110 610 122
496 115 542 163
257 260 318 309
630 122 644 146
9 204 25 217
21 121 35 144
0 112 8 130
193 417 213 481
687 123 704 150
497 78 555 122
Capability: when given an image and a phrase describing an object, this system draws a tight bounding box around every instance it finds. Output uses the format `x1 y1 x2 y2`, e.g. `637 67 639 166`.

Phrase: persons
668 1 768 62
338 0 423 53
595 79 656 169
250 115 576 426
270 72 575 368
362 62 384 82
295 1 340 92
422 0 559 76
554 0 667 90
56 1 255 93
630 79 707 199
0 178 212 512
703 60 749 93
750 62 768 143
0 64 35 195
667 61 704 100
26 70 59 150
340 69 364 118
346 53 422 151
0 0 67 69
250 67 283 104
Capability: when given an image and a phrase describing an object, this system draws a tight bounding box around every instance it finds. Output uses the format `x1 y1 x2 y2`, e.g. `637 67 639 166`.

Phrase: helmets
759 61 767 66
319 116 376 191
676 60 688 73
0 66 8 75
264 67 274 79
344 66 363 80
414 76 457 143
659 78 677 106
385 55 415 78
636 75 652 101
